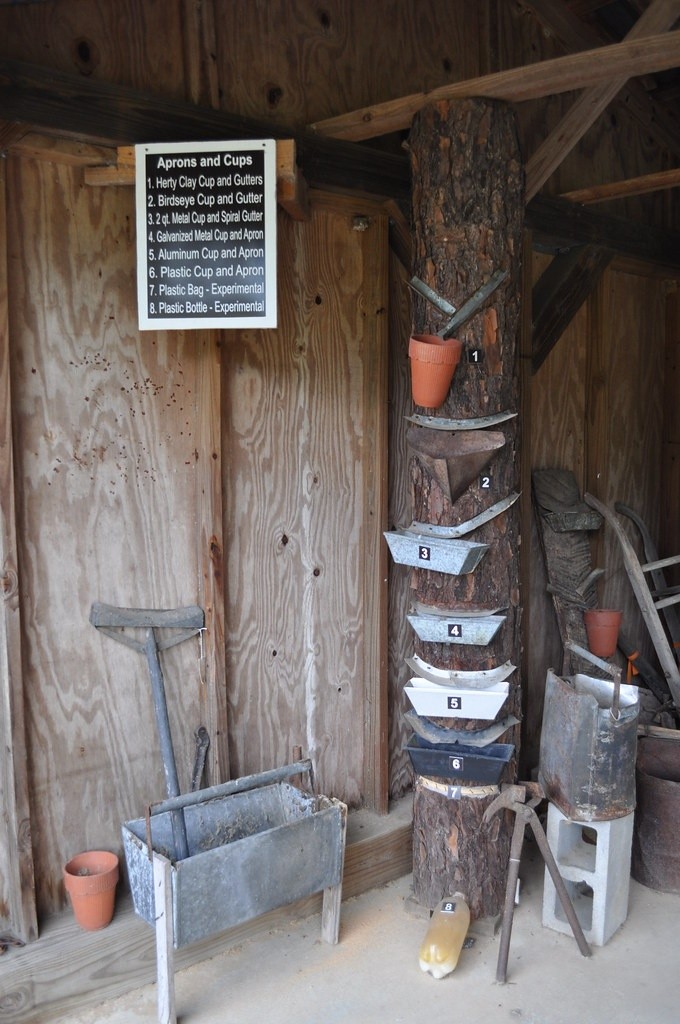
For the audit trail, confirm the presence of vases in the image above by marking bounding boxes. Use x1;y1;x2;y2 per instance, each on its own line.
405;427;505;507
408;335;463;409
403;677;510;720
406;611;508;646
545;511;604;532
401;733;516;785
583;608;624;657
382;530;490;575
62;850;120;931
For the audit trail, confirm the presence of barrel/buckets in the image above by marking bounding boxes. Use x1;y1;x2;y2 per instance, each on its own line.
536;637;643;822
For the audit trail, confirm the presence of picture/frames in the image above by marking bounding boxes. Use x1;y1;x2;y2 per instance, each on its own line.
134;139;281;332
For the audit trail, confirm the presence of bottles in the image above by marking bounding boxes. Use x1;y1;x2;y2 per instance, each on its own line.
418;891;471;979
